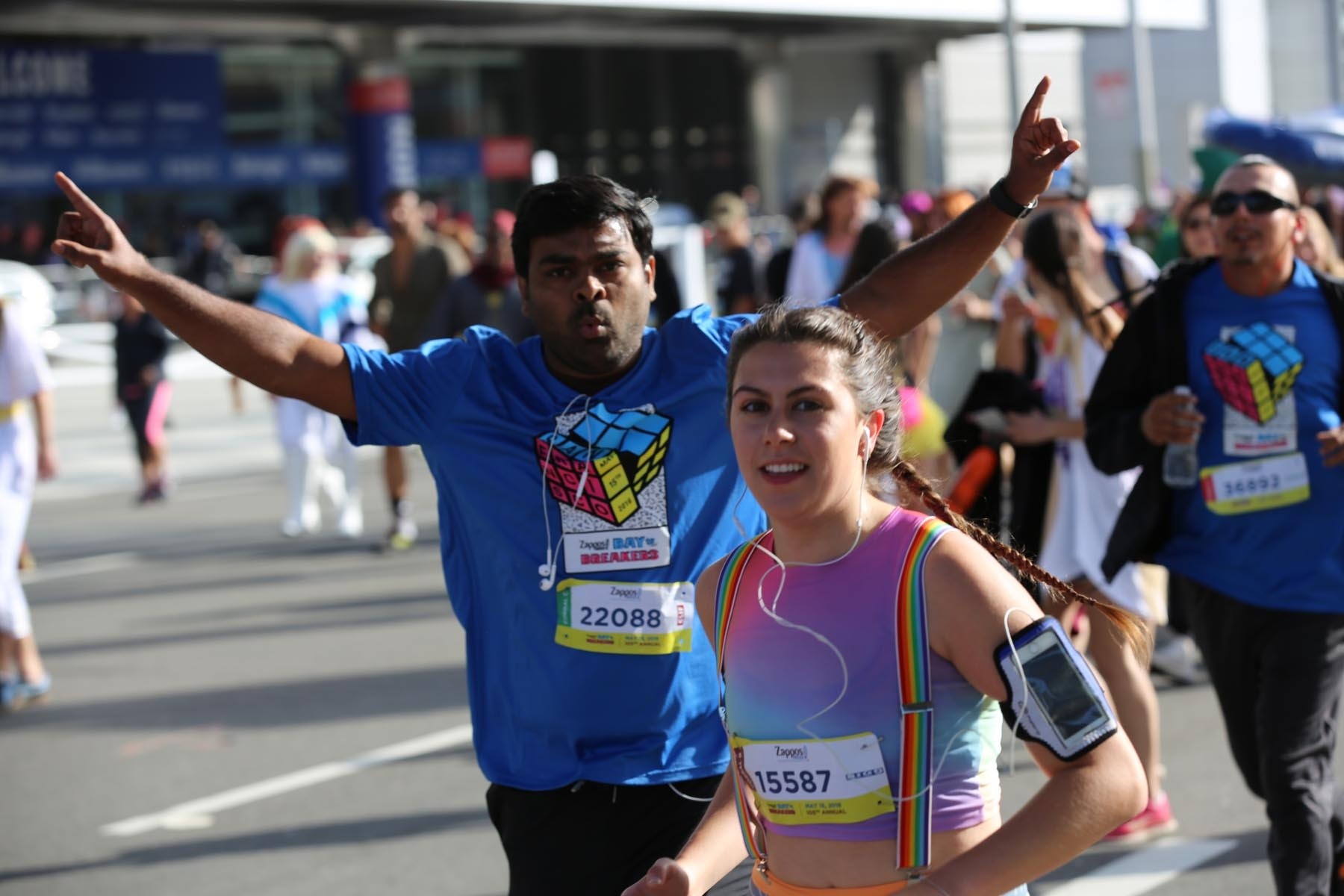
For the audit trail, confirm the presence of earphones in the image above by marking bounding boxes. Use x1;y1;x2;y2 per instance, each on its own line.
859;426;872;461
537;548;558;592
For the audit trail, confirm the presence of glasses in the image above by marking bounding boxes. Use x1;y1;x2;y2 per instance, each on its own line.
1209;189;1299;217
1183;217;1210;232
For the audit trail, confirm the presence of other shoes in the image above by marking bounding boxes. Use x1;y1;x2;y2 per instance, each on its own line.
1151;636;1208;682
1105;791;1178;843
138;482;165;504
339;513;365;537
388;517;419;551
283;510;321;537
1152;624;1182;649
1;674;50;714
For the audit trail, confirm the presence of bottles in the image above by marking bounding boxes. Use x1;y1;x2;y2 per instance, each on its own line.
1163;385;1197;487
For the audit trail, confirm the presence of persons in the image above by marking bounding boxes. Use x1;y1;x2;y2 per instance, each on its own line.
54;75;1079;896
619;307;1151;896
641;176;1344;854
113;292;173;502
188;190;540;548
0;259;57;712
1079;152;1344;896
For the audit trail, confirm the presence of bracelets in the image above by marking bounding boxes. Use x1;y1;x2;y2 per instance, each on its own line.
921;877;949;896
990;178;1038;219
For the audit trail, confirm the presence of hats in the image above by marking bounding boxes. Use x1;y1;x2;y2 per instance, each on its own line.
1038;165;1090;202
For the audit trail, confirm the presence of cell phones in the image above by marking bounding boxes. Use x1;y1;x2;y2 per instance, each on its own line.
1009;627;1112;749
